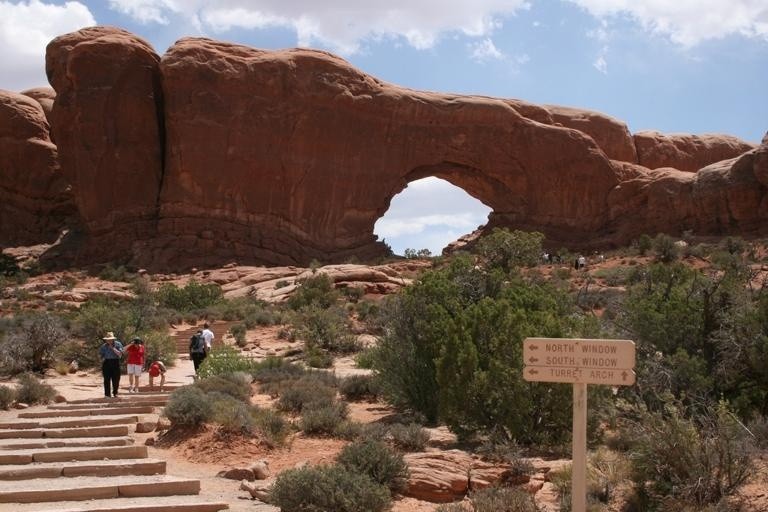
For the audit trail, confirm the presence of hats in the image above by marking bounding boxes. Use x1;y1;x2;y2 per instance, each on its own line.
130;337;143;344
150;365;159;377
102;332;117;341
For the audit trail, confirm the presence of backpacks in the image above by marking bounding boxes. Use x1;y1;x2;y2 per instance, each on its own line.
191;333;203;350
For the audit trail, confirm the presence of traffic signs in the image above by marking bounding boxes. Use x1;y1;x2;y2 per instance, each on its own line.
523;366;635;386
524;336;634;369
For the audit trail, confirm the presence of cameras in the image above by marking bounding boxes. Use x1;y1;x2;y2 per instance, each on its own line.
134;340;142;344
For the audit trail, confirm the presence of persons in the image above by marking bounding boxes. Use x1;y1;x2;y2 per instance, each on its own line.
123;337;146;393
189;330;209;372
202;322;215;358
542;251;601;268
97;331;124;399
148;361;166;392
